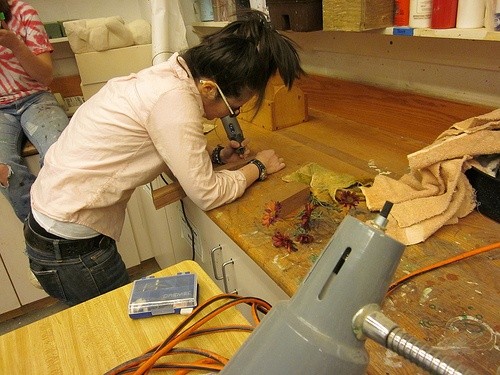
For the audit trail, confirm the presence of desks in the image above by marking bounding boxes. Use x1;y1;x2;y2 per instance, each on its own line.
0;259;255;375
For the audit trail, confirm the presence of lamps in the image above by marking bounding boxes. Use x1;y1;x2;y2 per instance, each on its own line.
218;200;481;375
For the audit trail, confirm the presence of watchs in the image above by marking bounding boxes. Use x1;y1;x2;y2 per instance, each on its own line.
247;159;267;182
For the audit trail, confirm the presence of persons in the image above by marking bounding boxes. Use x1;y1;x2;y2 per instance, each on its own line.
0;0;69;222
23;8;307;307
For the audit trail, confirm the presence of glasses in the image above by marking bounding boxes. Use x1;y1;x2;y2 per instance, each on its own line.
200;80;241;118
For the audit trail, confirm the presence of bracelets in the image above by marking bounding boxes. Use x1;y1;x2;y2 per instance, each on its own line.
211;146;225;165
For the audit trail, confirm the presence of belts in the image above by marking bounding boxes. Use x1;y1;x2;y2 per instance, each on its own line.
23;219;110;254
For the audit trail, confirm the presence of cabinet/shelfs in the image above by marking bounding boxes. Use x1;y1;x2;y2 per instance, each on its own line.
0;45;290;328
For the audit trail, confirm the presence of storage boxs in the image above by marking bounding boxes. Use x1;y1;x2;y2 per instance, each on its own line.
322;0;395;31
128;271;198;318
